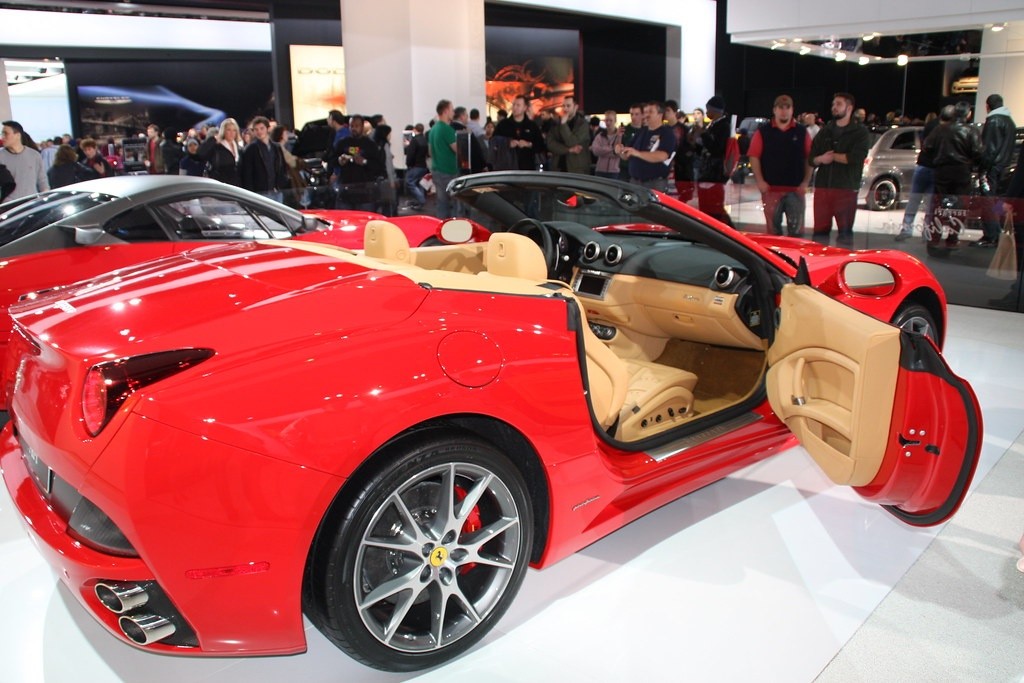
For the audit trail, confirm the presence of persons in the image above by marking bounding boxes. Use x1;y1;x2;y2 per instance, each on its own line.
746;95;814;239
923;101;984;249
809;92;868;250
895;105;954;243
0;94;749;227
969;94;1015;247
794;110;938;134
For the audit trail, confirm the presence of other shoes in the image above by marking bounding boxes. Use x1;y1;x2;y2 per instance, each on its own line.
413;204;425;210
928;239;940;247
895;229;912;241
946;240;961;249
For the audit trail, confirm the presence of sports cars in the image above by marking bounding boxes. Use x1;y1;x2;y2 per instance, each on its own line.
0;174;495;260
0;166;985;673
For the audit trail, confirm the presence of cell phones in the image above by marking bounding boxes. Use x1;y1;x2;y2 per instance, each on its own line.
621;122;624;132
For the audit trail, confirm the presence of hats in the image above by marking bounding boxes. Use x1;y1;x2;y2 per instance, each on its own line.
707;96;726;112
774;96;793;106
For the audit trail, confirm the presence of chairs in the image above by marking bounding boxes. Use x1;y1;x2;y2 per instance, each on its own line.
361;219;697;434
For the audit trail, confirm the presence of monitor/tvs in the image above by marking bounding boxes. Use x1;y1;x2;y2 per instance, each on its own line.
456;132;485;174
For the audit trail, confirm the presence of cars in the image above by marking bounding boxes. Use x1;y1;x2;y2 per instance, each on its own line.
952;75;979;96
740;117;771;137
486;63;574;116
857;126;928;211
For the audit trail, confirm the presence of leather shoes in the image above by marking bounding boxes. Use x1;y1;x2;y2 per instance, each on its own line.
970;238;997;247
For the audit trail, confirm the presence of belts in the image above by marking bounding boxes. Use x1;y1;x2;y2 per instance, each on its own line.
634;177;664;181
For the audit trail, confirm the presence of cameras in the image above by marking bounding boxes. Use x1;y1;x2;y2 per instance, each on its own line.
620;151;628;160
